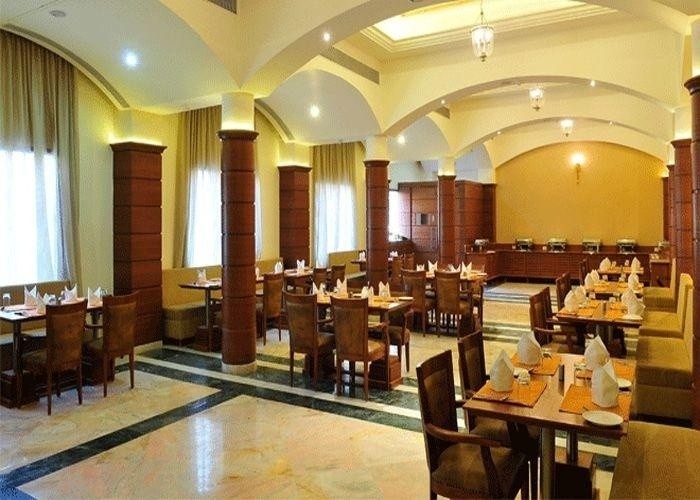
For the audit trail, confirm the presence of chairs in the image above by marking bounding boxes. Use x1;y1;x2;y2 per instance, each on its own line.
386;251;484;339
20;297;90;416
418;349;529;500
457;330;544;500
527;253;694;360
281;249;410;401
255;272;283;346
82;288;143;397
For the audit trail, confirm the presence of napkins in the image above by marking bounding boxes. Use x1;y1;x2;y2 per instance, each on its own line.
197;269;208;285
591;360;620;407
491;351;515;392
517;332;542;364
585;336;610;372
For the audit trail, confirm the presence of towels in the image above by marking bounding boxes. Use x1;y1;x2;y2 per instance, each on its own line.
88;286;101;306
64;284;77;297
24;284;37;303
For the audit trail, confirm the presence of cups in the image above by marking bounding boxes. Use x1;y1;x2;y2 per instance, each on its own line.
574;356;587;367
612;263;615;270
542;344;552;360
519;373;530;386
602;274;608;281
575;369;585;386
381;290;390;305
612;290;620;298
619;277;625;287
2;286;108;308
609;297;616;309
588;291;595;301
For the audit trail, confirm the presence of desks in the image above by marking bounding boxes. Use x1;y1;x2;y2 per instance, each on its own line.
178;276;263;351
463;353;637;500
0;298;103;409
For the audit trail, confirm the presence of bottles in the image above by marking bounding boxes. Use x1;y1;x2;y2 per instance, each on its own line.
602;300;607;310
558;361;565;381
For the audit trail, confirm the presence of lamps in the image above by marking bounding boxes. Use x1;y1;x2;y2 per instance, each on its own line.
528;82;546;111
469;0;497;64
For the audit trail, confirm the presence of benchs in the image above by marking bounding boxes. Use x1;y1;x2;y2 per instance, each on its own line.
0;279;99;398
631;289;695;422
608;424;699;500
161;266;222;345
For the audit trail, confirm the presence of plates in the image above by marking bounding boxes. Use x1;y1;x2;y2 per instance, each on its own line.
477;273;488;276
353;294;361;297
512;366;527;377
615;377;631;390
582;409;624;426
623;316;642;321
398;296;414;301
